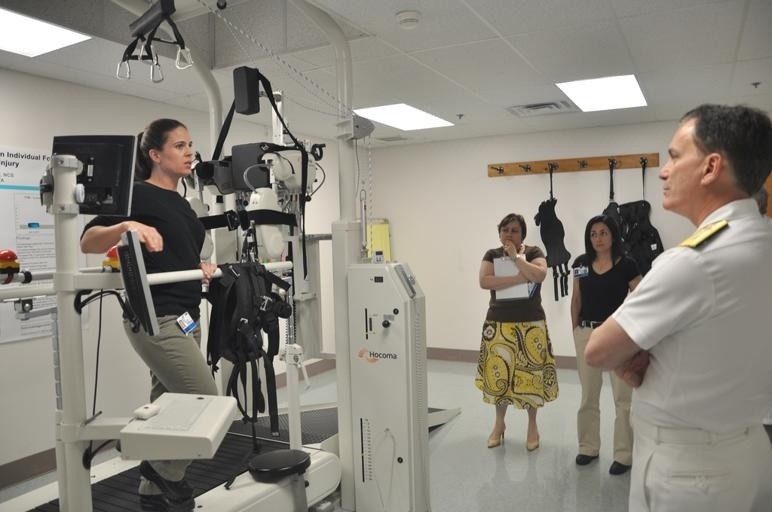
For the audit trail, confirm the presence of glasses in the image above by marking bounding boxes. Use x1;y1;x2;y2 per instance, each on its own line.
590;232;608;238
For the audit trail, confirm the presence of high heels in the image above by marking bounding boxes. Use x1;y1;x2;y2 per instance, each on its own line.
527;430;539;451
487;425;506;448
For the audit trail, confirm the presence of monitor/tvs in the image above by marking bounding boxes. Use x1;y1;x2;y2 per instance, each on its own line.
117;231;160;337
52;136;138;218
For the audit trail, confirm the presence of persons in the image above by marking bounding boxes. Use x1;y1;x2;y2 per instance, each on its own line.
584;104;772;512
80;118;218;512
571;215;643;474
479;213;559;450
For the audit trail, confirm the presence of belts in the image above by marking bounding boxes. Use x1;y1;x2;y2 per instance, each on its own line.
629;418;749;446
577;318;607;330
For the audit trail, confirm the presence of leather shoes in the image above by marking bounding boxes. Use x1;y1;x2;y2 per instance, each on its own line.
576;453;599;465
138;460;195;512
609;461;631;475
